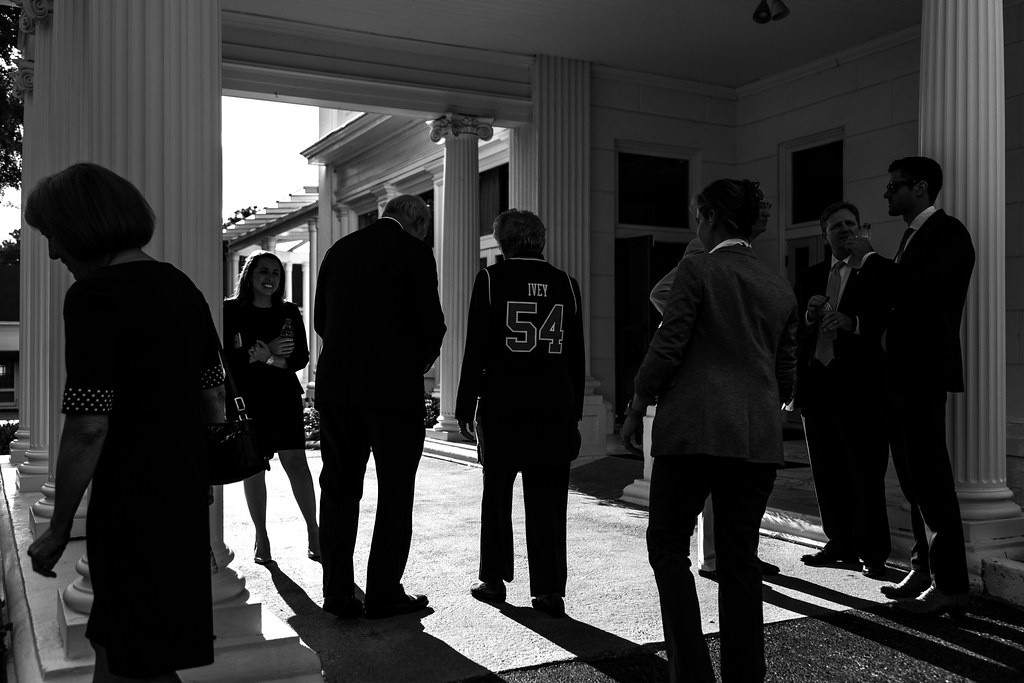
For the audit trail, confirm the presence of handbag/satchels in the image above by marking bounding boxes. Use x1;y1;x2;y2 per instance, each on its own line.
202;418;270;485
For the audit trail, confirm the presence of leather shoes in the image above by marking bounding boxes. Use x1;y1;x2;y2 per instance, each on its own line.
896;588;965;619
882;569;931;599
862;562;885;575
801;548;859;567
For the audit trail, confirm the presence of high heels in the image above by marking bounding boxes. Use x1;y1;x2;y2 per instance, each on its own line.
255;537;271;562
308;538;321;557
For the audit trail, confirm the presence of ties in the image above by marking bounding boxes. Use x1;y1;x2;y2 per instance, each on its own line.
897;228;915;263
814;261;846;366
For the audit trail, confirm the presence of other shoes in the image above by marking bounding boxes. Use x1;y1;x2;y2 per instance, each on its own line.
471;583;507;601
760;563;780;573
322;595;362;611
533;596;565;617
363;594;429;618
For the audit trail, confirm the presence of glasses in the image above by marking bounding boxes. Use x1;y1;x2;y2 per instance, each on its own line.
887;181;920;191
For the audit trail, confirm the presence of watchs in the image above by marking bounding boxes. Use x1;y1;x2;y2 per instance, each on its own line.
624;399;647;417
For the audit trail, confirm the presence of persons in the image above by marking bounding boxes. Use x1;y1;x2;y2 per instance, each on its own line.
223;252;320;563
25;160;226;683
792;156;975;613
314;194;447;620
455;208;587;616
621;181;798;683
